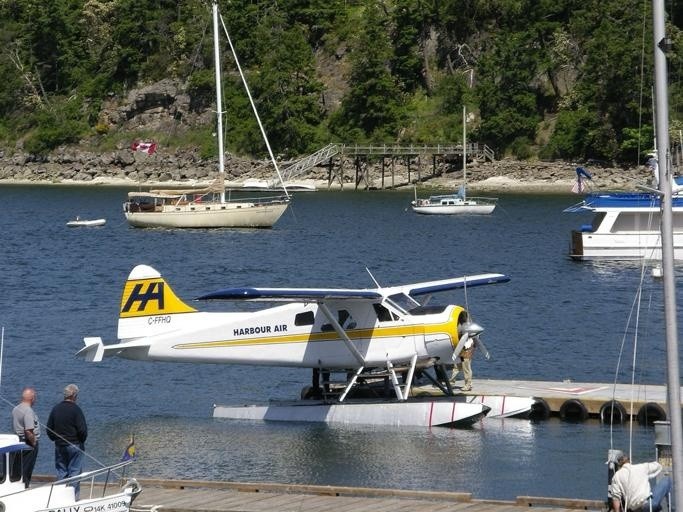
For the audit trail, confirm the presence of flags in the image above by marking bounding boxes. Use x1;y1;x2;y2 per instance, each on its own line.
130;140;156;155
570;175;585;194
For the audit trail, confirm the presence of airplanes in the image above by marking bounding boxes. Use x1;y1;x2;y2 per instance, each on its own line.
75;265;537;428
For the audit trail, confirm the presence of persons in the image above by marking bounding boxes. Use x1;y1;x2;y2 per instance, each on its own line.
606;448;673;512
9;384;91;499
449;333;475;391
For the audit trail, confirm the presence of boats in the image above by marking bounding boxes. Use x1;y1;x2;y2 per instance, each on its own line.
411;105;499;214
66;218;106;228
122;4;291;228
0;425;143;512
563;177;683;262
